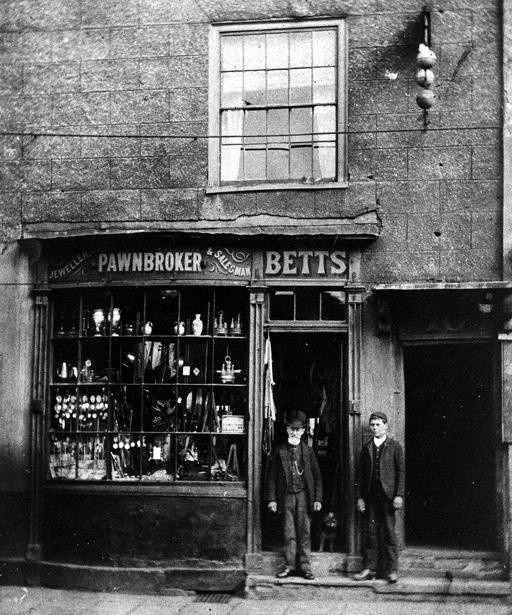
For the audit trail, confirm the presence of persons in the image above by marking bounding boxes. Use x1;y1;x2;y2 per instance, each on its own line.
263;410;324;580
353;410;406;583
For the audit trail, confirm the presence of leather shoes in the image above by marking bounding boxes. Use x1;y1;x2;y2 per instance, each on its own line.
354;569;398;583
277;568;315;579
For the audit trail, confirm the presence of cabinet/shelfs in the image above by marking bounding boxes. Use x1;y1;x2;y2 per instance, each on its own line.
45;286;250;487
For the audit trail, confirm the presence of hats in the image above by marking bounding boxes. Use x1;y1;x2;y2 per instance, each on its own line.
287;410;307;428
370;413;386;419
